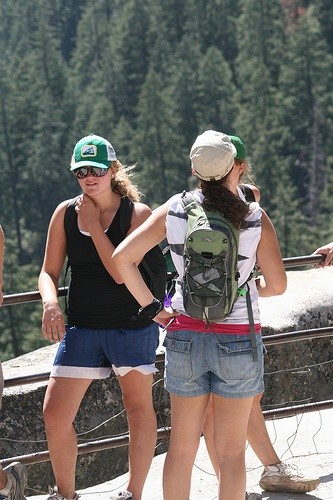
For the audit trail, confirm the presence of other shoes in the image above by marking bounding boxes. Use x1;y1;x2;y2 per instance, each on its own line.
245;492;261;500
0;462;27;500
46;487;80;500
108;490;132;500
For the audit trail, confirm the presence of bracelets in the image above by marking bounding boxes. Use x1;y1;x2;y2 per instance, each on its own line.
138;298;164;322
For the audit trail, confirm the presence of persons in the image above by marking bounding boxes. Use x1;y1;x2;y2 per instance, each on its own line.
112;130;320;500
38;133;166;500
0;226;27;500
311;242;333;268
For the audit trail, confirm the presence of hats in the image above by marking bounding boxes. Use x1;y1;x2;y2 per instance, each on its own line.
68;134;116;169
189;130;244;181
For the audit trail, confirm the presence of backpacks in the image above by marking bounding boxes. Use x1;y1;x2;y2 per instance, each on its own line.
178;191;258;365
64;194;169;309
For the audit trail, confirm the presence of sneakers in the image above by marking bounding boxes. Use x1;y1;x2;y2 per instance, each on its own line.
260;462;320;494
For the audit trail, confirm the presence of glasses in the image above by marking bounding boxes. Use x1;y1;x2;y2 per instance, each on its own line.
73;166;111;179
